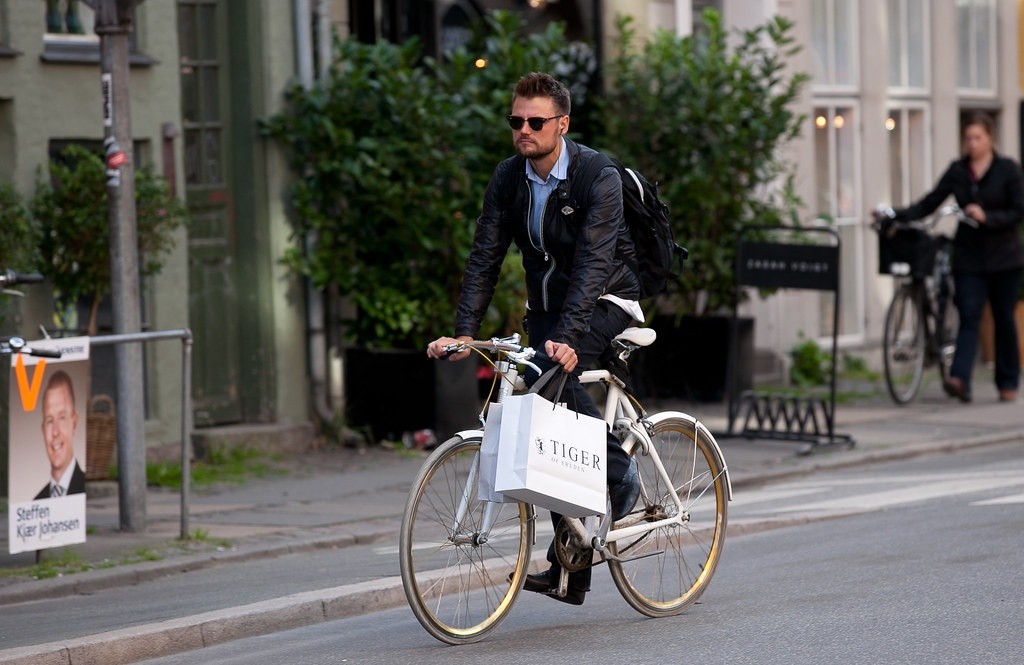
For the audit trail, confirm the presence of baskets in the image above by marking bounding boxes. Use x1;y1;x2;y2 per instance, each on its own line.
879;222;932;276
86;395;118;479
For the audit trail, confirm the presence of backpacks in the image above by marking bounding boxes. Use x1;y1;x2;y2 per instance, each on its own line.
510;154;688;299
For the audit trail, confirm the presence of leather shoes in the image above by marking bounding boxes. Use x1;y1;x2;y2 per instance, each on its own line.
609;459;640;521
510;566;585;606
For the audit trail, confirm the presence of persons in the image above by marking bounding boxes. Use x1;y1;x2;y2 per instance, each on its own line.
428;70;642;606
34;370;84;500
874;113;1024;400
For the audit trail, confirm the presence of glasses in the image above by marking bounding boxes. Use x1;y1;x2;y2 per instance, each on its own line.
505;113;563;131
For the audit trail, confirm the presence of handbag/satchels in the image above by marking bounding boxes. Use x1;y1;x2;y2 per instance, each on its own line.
478;362;610;518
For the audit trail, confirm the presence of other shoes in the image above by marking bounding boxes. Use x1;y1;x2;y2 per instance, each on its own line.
946;378;971;402
1000;389;1016;404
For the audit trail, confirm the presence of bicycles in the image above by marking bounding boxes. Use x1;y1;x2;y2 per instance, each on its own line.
399;327;734;645
870;203;959;407
0;270;62;358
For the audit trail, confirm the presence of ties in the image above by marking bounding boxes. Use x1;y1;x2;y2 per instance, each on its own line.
49;485;65;498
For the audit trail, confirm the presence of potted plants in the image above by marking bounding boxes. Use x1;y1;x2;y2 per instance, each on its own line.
595;4;815;407
257;42;503;442
